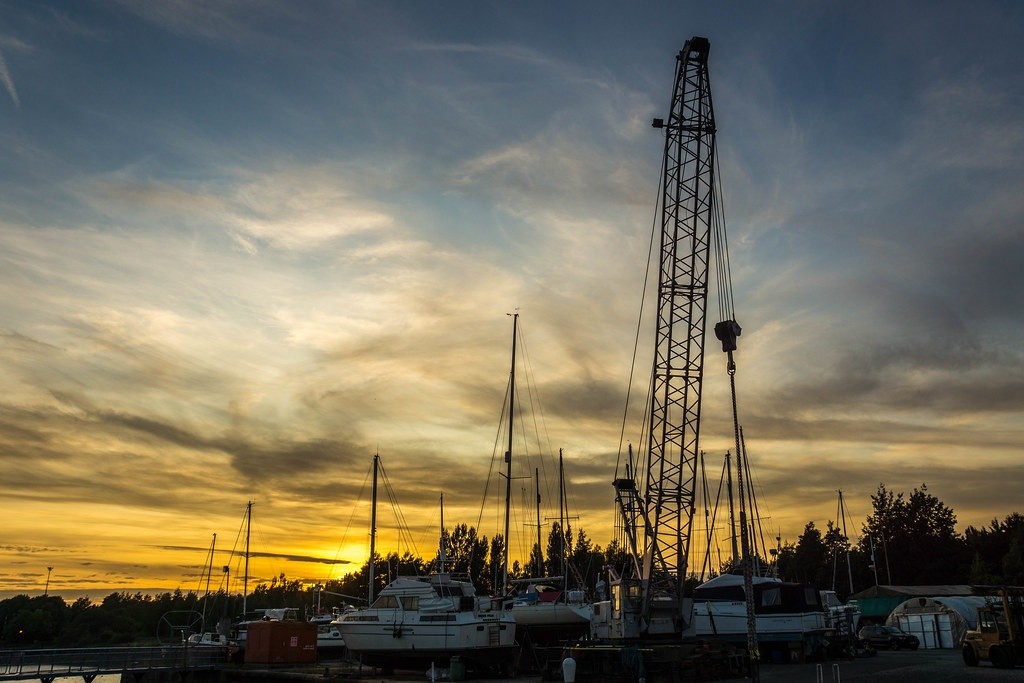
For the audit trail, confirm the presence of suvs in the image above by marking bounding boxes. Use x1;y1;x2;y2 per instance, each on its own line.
858;624;920;652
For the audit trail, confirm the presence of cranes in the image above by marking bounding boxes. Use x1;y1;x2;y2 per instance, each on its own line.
612;34;749;626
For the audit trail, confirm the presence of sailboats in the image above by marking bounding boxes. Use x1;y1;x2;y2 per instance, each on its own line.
182;308;849;661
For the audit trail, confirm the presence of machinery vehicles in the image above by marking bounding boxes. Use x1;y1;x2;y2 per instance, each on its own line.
957;583;1024;670
